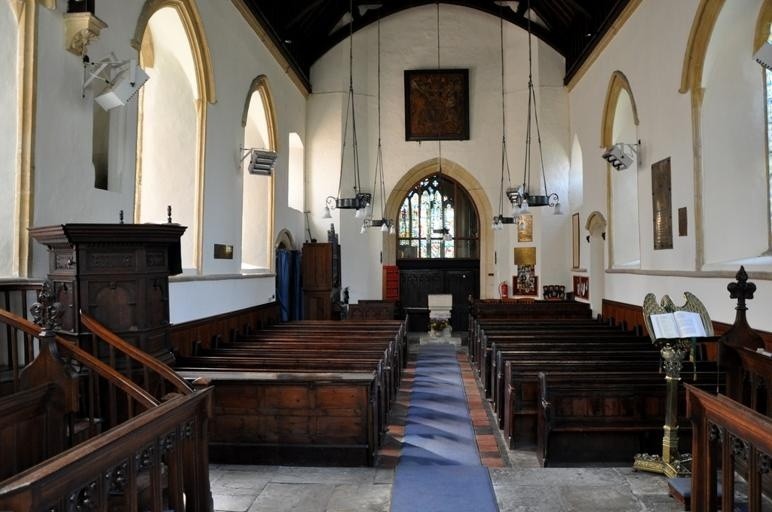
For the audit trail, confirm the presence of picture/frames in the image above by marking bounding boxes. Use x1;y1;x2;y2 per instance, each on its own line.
402;67;469;140
649;156;675;251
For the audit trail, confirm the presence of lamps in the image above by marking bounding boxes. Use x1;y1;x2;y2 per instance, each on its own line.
506;186;524;207
602;142;641;171
511;0;564;216
83;57;151;114
321;1;371;219
239;145;277;177
360;0;397;236
491;0;526;231
426;1;452;241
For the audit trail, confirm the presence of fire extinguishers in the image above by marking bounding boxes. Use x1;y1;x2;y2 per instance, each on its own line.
498;281;509;298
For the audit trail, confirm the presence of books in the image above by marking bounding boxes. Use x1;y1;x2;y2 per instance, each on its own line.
649;310;707;340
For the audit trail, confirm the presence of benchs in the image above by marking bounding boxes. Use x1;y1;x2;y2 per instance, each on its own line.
537;372;717;467
159;368;383;467
504;360;716;450
496;349;697;430
479;323;643;391
490;340;709;414
468;313;603;364
471;315;615;369
274;318;408;352
250;329;404;377
208;346;393;426
260;323;406;370
473;320;627;375
222;341;397;410
187;356;391;449
238;335;401;397
483;334;652;400
